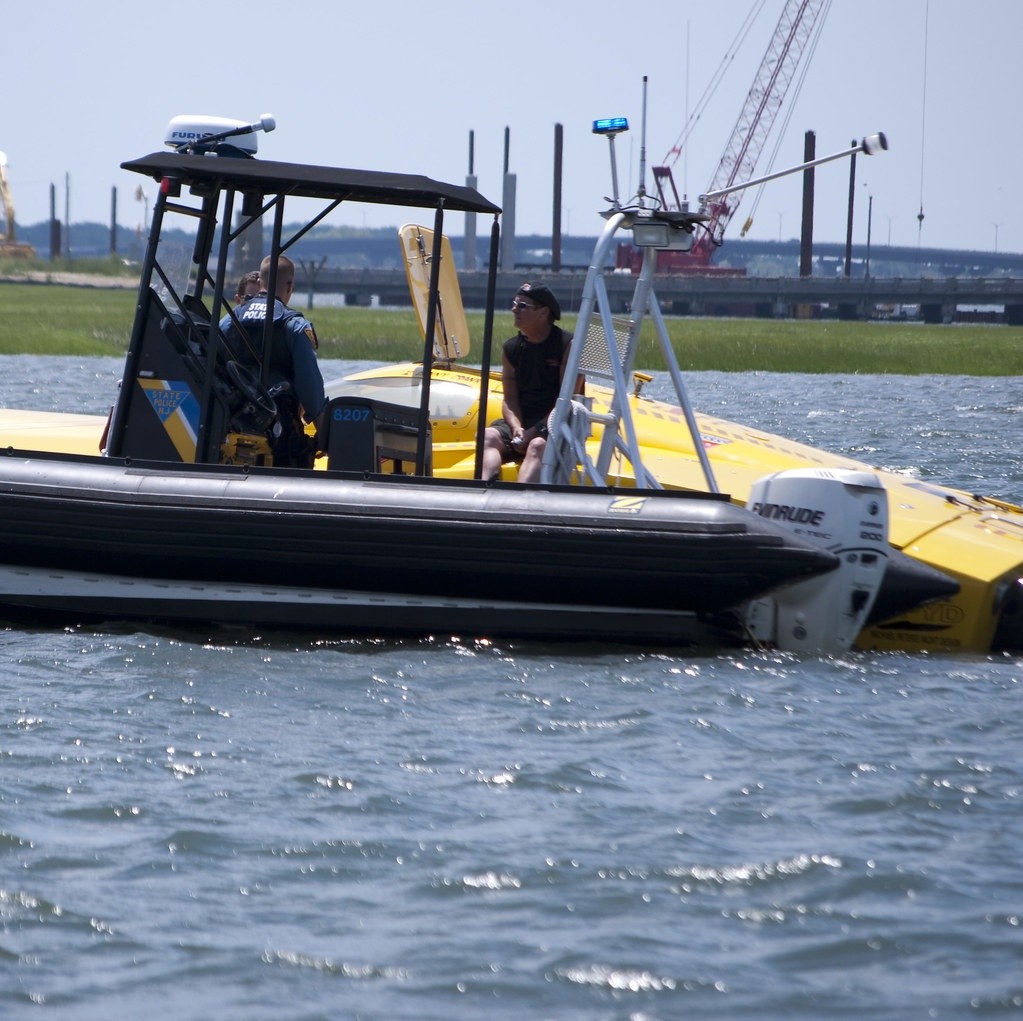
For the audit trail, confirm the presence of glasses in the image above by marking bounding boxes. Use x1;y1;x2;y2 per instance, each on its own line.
240;294;253;301
512;301;540;311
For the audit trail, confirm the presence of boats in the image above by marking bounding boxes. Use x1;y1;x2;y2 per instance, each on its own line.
0;222;1022;654
0;77;962;659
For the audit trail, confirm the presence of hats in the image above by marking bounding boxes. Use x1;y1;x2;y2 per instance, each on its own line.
517;281;561;321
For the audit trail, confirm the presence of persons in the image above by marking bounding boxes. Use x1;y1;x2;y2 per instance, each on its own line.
232;254;327;469
217;271;261;346
475;281;575;482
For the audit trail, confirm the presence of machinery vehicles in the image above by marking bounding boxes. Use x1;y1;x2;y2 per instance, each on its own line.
611;0;835;275
